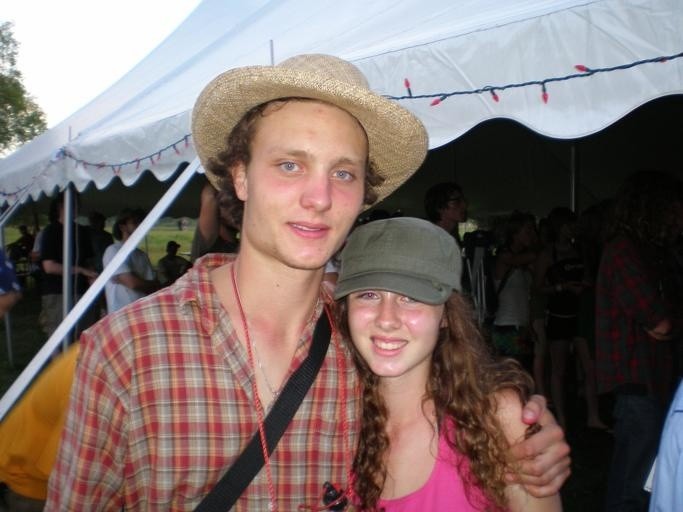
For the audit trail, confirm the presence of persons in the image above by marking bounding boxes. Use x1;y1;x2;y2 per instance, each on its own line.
41;52;575;512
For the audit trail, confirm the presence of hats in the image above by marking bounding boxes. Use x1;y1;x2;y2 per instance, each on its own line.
189;54;429;214
332;217;463;306
